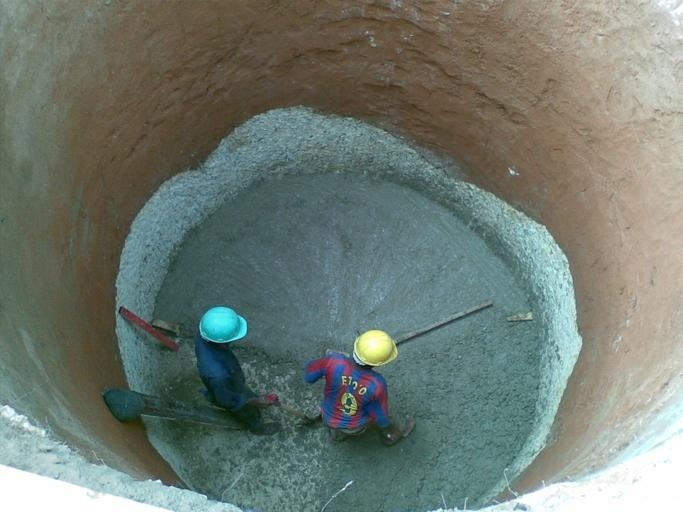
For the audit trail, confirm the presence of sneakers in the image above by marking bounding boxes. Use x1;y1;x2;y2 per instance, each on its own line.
251;423;279;436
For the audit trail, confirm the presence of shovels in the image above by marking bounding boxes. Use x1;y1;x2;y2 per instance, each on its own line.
264;394;323;427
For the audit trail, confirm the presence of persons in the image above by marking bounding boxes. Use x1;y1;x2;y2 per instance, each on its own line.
193;306;279;436
303;329;415;445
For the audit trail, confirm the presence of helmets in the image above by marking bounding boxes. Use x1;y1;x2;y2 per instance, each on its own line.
199;307;247;344
354;330;398;366
